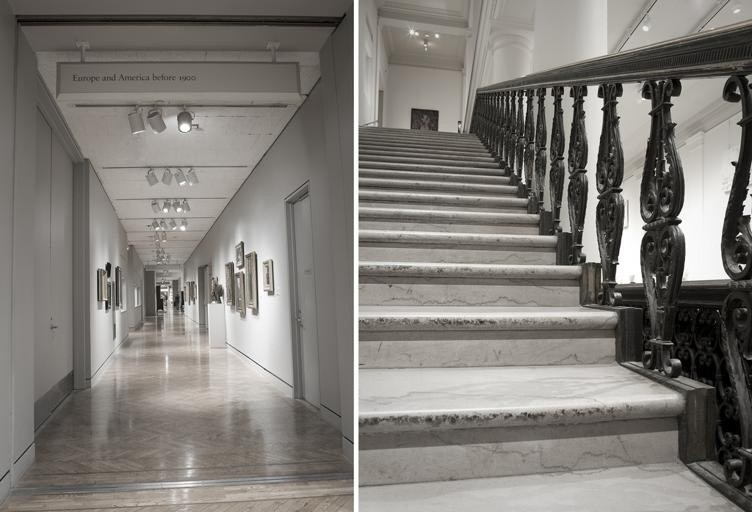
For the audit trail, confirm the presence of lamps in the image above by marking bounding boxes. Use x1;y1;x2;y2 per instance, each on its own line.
151;199;191;215
126;103;200;137
145;168;199;187
148;218;188;244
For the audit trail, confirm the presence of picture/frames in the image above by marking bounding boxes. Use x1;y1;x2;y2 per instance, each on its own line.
224;241;258;319
97;262;123;311
261;258;274;292
410;107;440;131
